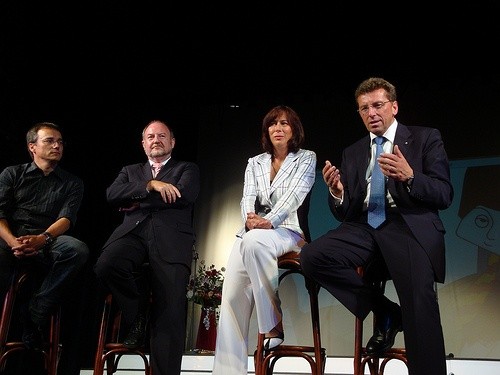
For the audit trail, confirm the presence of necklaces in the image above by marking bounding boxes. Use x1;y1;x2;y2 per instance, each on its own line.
271;162;276;174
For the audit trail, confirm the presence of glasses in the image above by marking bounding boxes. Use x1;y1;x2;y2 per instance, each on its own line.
357;101;392;111
42;139;62;146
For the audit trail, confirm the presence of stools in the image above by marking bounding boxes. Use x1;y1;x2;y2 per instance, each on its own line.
0;259;62;375
254;251;328;375
351;257;409;375
93;262;159;375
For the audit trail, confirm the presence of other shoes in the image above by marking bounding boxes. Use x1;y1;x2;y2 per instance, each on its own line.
261;321;283;350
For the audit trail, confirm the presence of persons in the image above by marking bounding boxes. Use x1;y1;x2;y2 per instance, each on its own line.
300;77;455;375
96;119;197;375
212;106;316;375
0;122;88;352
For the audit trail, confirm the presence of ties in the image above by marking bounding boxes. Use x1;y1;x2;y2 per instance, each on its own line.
368;137;388;229
153;163;163;178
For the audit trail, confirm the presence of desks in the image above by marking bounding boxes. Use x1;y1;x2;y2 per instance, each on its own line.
195;303;217;353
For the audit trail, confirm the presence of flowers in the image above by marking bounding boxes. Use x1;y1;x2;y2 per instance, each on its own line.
186;260;224;331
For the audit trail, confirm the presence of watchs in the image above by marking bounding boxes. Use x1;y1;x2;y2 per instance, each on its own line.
405;177;414;188
42;231;54;244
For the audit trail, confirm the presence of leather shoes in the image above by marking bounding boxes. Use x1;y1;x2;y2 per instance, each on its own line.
123;321;149;346
366;303;402;355
23;319;45;350
